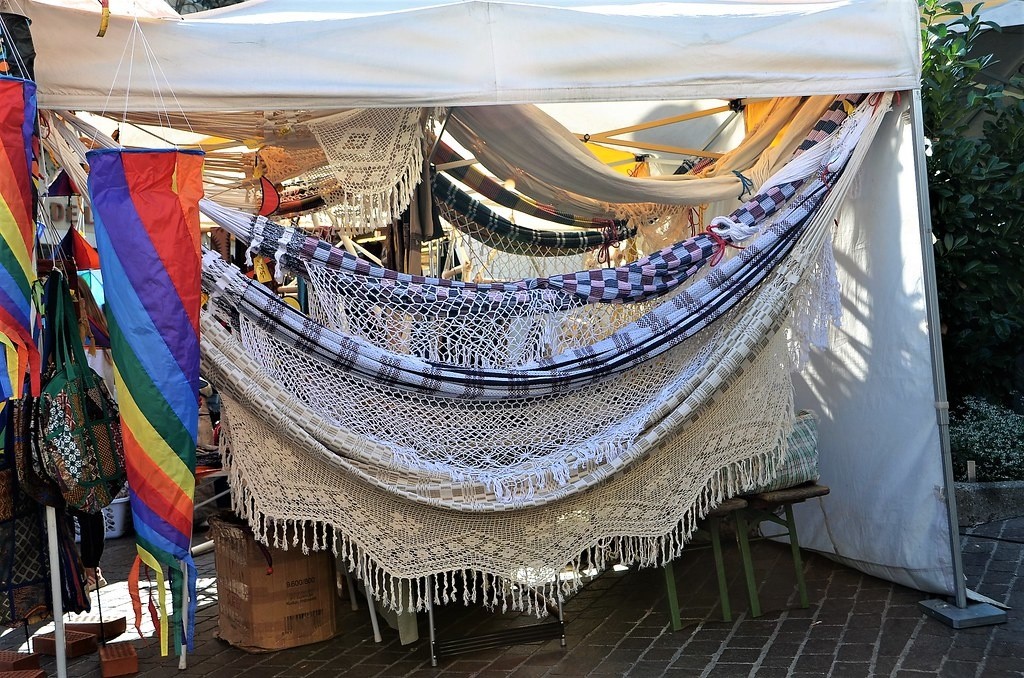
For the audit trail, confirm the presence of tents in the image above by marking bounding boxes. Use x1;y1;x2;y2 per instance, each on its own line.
0;0;973;607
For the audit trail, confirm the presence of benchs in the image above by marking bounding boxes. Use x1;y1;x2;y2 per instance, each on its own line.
645;485;830;633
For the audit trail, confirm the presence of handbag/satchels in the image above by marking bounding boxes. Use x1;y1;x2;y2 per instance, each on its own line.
707;409;821;495
11;269;126;620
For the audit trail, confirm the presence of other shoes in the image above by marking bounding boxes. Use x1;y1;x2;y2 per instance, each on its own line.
86;575;107;590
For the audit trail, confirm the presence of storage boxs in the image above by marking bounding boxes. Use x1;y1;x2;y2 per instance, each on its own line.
72;496;131;543
206;518;339;654
